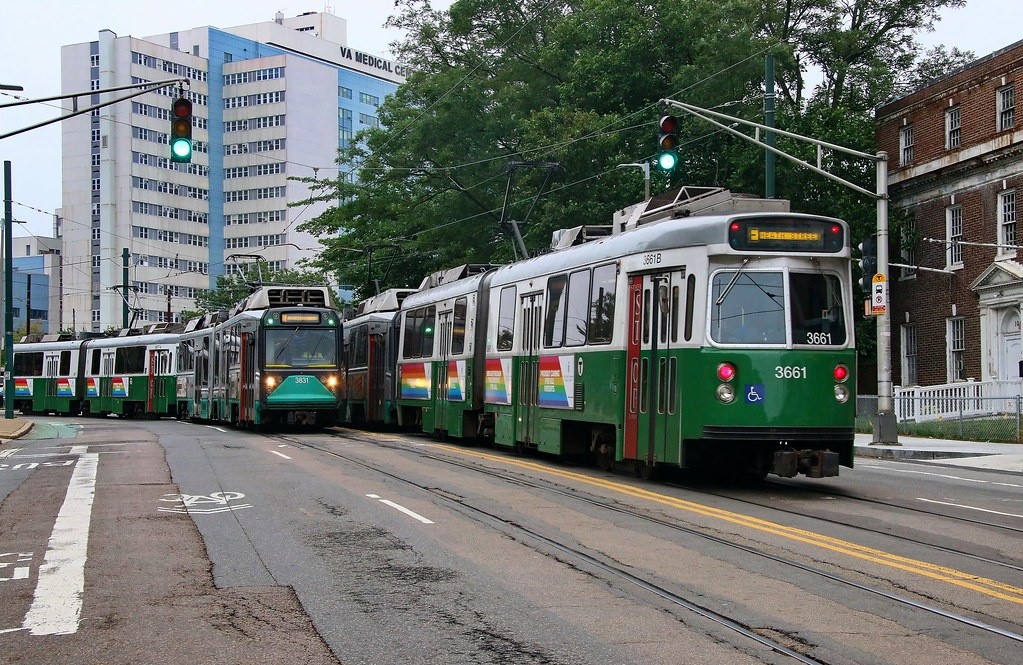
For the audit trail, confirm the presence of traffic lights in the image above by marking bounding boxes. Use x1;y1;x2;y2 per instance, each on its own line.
656;114;681;172
858;237;877;292
169;97;194;164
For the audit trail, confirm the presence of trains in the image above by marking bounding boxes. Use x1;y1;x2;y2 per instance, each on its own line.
0;282;348;433
341;183;856;488
